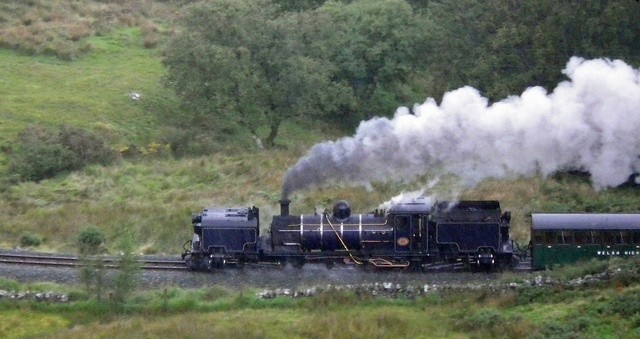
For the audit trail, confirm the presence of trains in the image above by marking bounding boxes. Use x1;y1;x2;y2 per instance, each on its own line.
181;195;639;274
